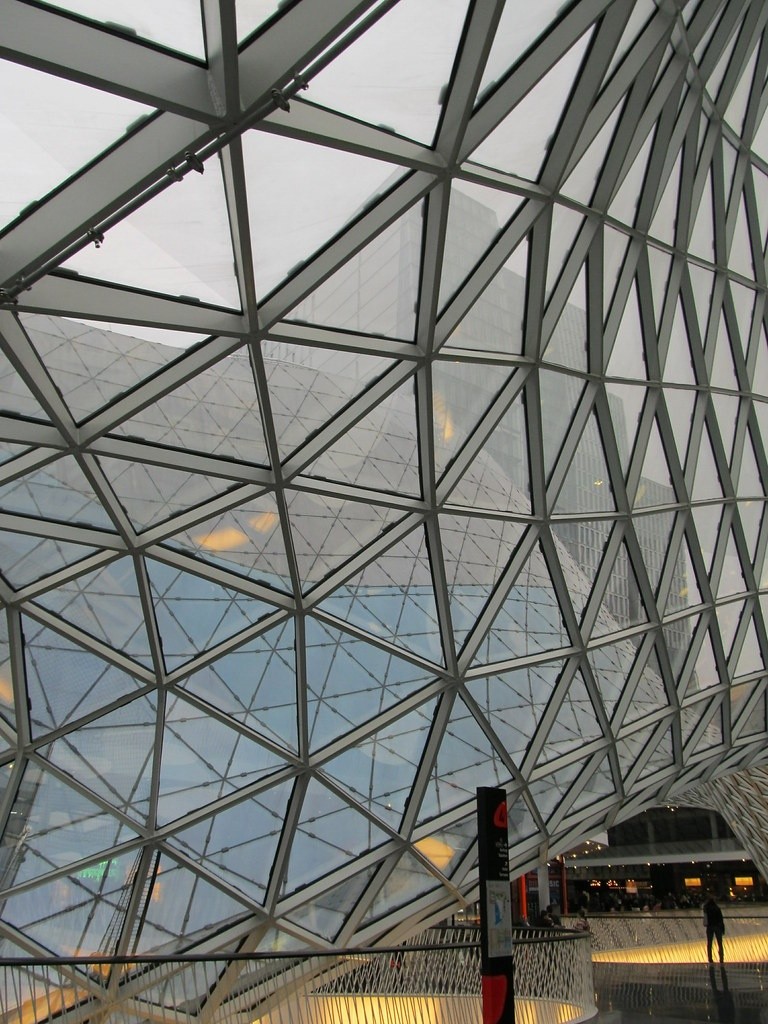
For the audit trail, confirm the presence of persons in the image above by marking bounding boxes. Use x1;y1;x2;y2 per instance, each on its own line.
532;905;563;928
577;889;705;911
703;896;725;963
574;910;590;931
